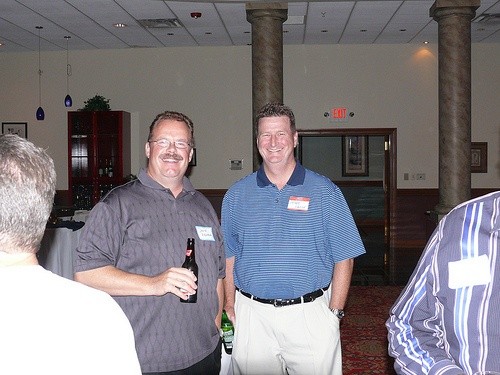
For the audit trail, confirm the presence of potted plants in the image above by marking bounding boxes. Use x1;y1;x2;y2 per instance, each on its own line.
78;95;110;112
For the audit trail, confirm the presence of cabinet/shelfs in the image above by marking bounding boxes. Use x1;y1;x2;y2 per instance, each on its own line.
67;110;132;209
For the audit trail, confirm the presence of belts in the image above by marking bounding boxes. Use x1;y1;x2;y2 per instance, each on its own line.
235;283;330;307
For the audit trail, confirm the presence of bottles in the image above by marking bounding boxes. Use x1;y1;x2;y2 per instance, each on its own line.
98;159;113;178
221;309;236;356
180;238;199;303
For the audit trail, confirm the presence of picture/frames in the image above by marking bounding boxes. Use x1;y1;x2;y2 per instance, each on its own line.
341;136;370;177
1;122;28;139
471;141;488;173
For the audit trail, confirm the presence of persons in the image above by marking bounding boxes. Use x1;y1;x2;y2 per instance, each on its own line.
384;191;500;375
220;101;366;375
73;111;226;375
0;134;142;375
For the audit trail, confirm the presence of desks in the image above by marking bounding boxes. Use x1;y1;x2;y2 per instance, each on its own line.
37;216;84;280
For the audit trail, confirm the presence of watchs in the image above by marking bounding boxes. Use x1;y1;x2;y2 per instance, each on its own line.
329;307;345;319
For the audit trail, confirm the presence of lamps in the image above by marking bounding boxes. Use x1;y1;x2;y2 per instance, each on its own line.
64;36;73;107
324;107;355;123
35;26;45;121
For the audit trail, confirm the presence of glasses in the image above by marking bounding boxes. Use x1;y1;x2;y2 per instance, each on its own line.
149;139;190;149
